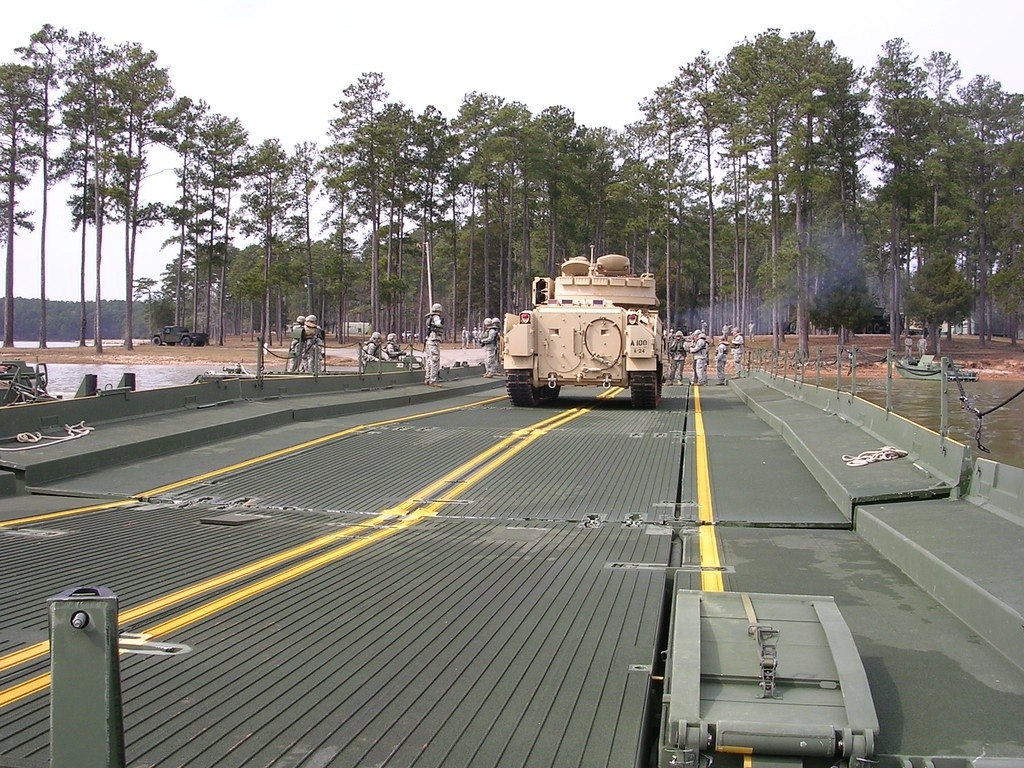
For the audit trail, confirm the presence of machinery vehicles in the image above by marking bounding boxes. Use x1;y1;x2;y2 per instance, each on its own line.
909;319;943;335
857;306;904;333
502;243;668;408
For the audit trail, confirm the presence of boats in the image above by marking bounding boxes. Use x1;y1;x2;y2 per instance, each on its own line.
895;356;979;382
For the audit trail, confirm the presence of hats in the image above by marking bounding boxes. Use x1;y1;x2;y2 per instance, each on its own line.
669;329;674;332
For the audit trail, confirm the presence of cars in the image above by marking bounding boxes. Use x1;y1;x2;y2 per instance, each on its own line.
401;331;418;339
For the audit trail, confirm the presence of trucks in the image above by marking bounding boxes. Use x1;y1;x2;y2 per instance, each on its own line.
341;322;373;335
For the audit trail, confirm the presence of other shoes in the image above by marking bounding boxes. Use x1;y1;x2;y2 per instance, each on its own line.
715;382;724;386
693;382;706;386
430;382;442;387
664;380;673;386
677;379;683;386
479;372;493;378
730;374;741;379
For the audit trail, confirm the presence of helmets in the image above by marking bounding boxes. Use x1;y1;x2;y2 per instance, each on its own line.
483;318;492;325
492;318;500;323
306;315;317;322
733;327;740;332
693;330;702;335
432;303;442;311
697;334;707;339
387;333;397;340
372;332;381;338
296;316;306;323
675;331;683;337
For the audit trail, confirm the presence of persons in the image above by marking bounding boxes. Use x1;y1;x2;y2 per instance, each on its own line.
904;334;913;359
747;320;756;342
461;318;503;378
662;320;744;387
385;333;406;361
917;335;927;358
361;332;386;370
286;314;323;373
424;303;444;387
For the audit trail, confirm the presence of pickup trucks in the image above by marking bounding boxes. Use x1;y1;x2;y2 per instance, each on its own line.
151;325;207;347
